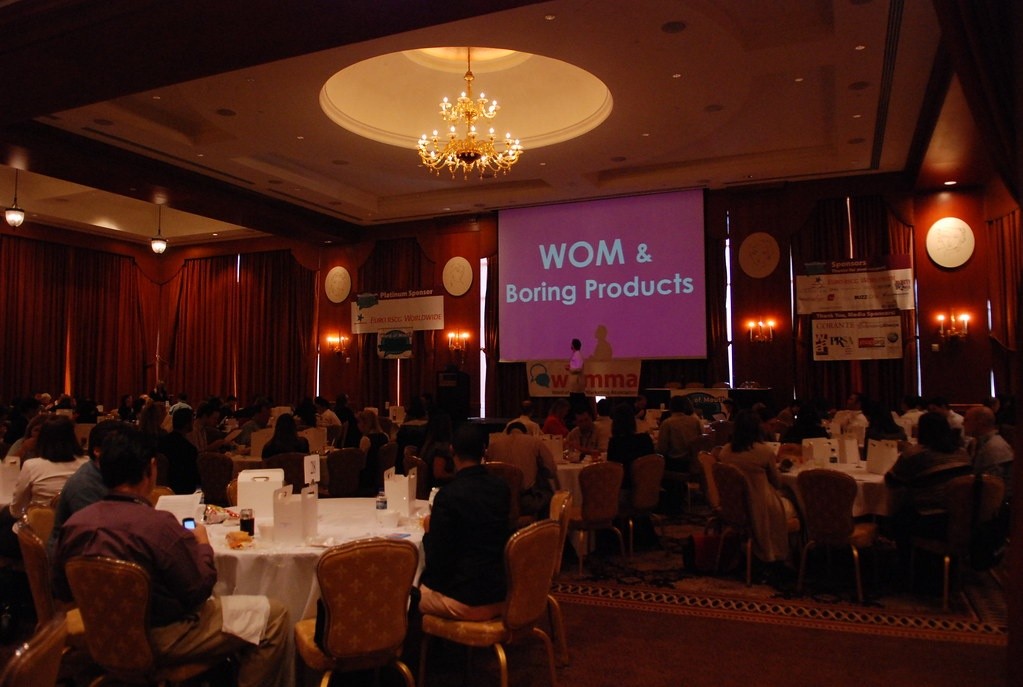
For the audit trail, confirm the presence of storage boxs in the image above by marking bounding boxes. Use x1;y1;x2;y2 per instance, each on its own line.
0;455;21;483
831;433;861;464
272;485;319;544
866;439;900;474
237;469;285;518
801;437;827;467
539;434;563;465
383;465;417;517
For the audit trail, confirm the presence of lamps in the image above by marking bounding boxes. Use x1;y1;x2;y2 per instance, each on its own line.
939;313;968;354
448;332;470;368
326;335;347;361
151;206;169;255
416;47;524;181
5;169;25;232
748;319;774;355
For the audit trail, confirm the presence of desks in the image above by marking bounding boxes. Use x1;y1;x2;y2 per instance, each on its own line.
201;497;430;687
557;453;602;558
223;447;340;488
779;461;892;519
0;470;16;554
644;387;777;419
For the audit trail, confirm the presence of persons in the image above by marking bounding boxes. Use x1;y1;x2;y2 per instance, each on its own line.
564;339;596;422
45;428;241;664
54;420;137;605
410;418;511;623
0;380;1013;646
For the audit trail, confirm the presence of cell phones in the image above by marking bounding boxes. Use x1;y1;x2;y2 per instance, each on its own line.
182;517;196;529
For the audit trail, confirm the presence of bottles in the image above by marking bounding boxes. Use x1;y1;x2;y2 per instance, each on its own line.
375;489;388;526
828;447;839;469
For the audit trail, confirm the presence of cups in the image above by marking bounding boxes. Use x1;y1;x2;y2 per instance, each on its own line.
239;508;255;538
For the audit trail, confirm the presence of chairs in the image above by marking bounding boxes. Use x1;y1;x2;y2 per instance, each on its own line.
0;405;1013;687
685;382;704;388
664;382;682;389
712;382;730;388
739;381;762;388
